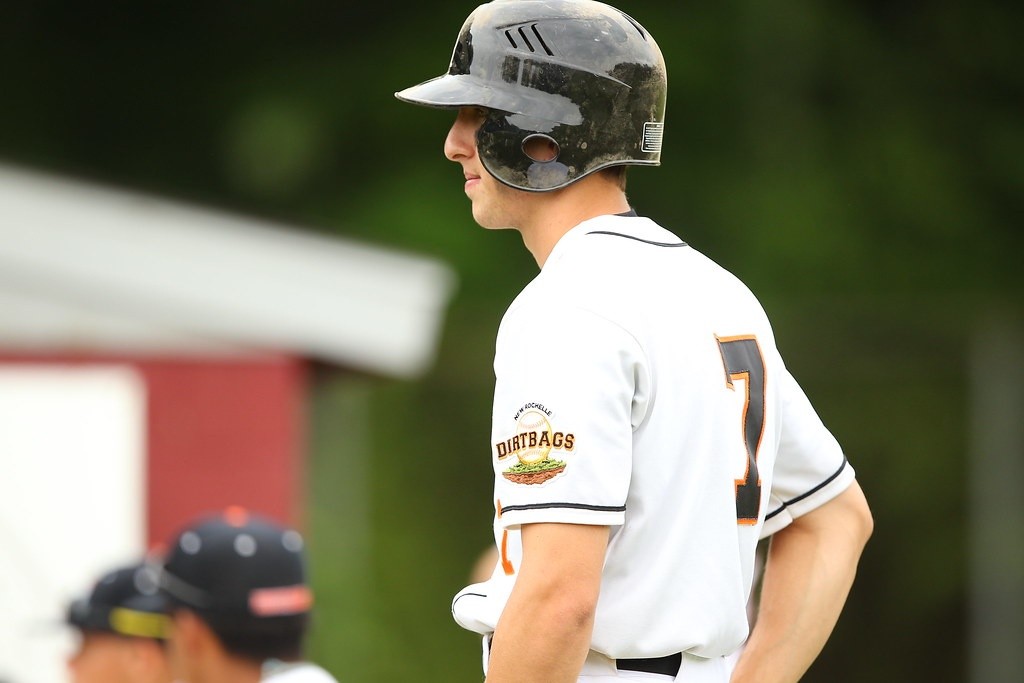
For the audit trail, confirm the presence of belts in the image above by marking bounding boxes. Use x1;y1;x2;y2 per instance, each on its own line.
488;631;682;678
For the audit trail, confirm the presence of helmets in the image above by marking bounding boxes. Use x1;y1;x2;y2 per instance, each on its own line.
394;0;668;193
156;506;316;631
63;563;175;641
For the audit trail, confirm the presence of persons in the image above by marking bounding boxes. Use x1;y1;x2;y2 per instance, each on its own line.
395;0;873;683
66;512;341;683
